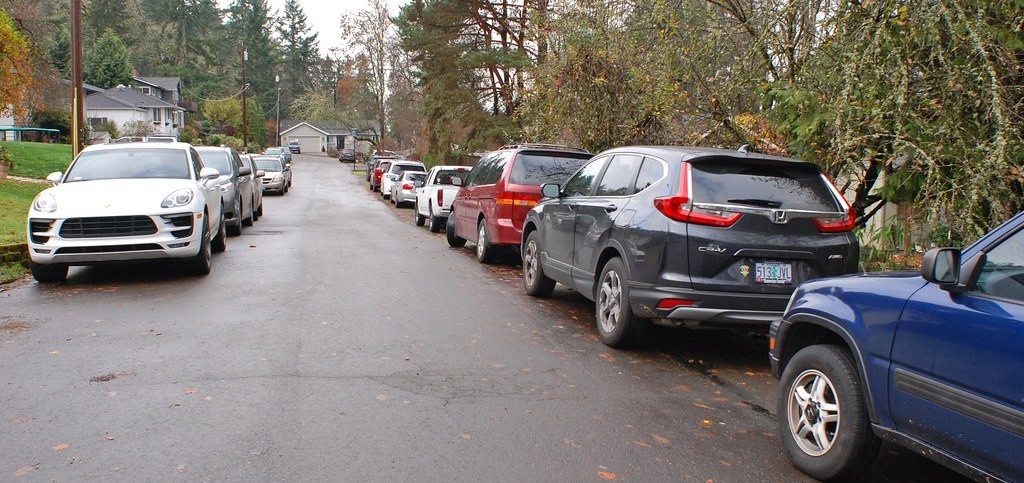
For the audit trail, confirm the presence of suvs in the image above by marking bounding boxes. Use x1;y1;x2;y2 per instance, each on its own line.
444;143;600;264
288;141;301;154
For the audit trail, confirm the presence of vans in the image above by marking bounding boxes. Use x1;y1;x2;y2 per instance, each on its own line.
113;135;179;143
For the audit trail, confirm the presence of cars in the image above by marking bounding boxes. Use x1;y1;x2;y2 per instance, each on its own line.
340;149;356;163
26;142;228;284
191;144;255;237
379;160;427;200
764;206;1024;483
362;149;400;193
239;154;266;220
252;146;292;196
520;144;860;350
389;170;430;208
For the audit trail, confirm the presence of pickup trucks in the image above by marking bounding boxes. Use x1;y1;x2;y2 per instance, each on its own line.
411;166;474;232
